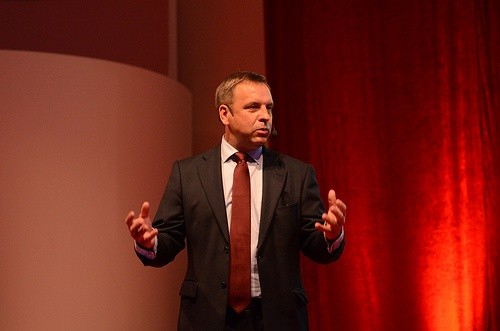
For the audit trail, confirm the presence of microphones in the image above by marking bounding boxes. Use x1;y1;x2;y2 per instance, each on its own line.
272;125;278;136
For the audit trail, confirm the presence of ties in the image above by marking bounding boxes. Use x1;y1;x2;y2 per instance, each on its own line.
227;152;255;313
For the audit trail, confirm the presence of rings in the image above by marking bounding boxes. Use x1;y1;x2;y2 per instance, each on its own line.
141;237;147;243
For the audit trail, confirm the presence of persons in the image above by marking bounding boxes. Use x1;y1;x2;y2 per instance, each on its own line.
125;72;348;331
